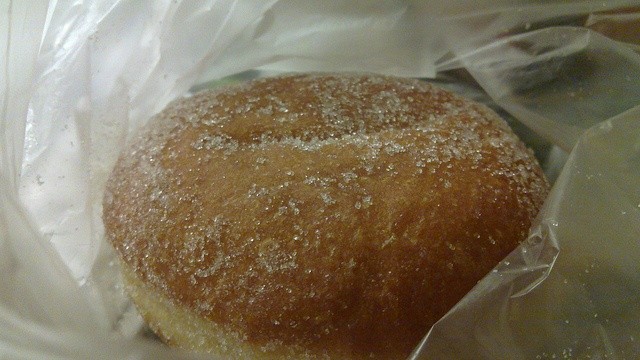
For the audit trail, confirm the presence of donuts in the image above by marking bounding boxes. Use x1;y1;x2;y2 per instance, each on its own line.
101;74;553;360
